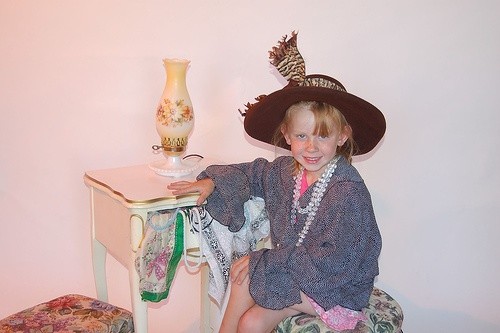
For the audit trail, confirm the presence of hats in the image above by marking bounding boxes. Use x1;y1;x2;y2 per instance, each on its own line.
238;31;386;156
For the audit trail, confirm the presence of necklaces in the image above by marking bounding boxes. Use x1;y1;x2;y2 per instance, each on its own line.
289;160;337;246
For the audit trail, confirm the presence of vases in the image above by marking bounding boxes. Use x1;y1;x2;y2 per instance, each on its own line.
149;58;198;178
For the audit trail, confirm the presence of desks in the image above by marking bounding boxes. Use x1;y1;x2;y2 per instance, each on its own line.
84;161;213;333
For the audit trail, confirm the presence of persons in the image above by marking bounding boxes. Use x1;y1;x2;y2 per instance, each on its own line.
168;73;381;333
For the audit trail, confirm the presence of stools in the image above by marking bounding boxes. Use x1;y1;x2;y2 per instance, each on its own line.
271;287;405;333
0;293;134;333
